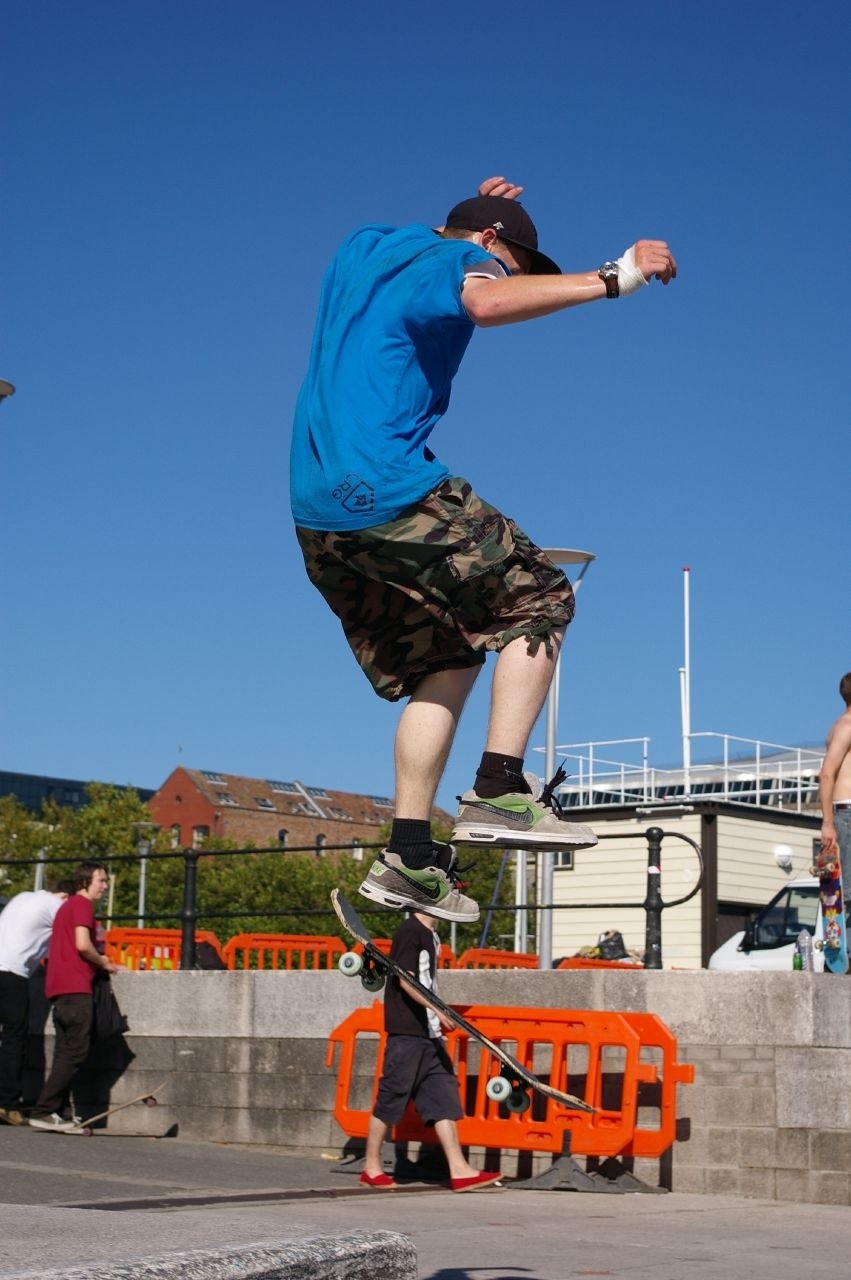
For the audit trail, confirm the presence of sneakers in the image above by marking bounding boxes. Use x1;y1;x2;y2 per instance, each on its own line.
451;757;598;851
28;1113;77;1132
358;838;481;923
61;1117;93;1136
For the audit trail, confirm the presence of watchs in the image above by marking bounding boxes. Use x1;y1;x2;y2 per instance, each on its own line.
598;260;620;299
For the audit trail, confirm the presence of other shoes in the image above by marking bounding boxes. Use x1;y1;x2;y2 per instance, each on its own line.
360;1170;396;1189
452;1171;505;1192
0;1105;30;1126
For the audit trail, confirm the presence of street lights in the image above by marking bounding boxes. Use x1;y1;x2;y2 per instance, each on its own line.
127;822;162;930
537;544;594;969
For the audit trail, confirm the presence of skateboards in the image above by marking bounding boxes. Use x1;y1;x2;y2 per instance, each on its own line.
53;1081;169;1138
326;886;596;1114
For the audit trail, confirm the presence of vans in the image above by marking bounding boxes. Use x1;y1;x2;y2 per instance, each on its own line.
707;876;842;974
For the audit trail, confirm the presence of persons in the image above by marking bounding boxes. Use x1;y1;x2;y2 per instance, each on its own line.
818;672;851;972
0;860;122;1135
358;911;507;1194
289;175;678;924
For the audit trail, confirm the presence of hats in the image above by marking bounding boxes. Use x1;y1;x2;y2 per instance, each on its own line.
446;195;563;274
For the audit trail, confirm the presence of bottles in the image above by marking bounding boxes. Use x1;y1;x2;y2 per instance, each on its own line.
793;952;801;971
797;927;814;970
